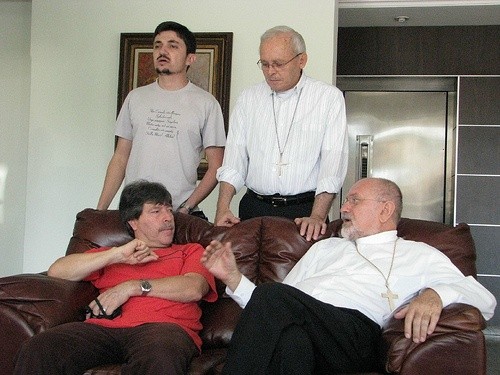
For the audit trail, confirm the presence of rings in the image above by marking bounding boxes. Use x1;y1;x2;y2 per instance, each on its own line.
103;309;107;312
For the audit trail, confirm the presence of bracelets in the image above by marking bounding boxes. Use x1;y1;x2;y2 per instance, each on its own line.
179;203;193;214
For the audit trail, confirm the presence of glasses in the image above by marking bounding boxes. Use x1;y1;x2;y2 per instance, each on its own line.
256;52;304;71
343;197;386;208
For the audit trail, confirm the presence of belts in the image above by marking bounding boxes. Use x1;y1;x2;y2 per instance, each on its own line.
246;187;315;208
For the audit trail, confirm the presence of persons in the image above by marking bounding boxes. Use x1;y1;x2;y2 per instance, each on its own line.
10;178;218;375
96;21;227;214
200;177;497;375
214;25;348;241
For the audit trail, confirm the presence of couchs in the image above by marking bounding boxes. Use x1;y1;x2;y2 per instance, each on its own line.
0;207;487;375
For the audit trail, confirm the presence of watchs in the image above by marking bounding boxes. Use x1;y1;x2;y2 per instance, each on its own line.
140;279;152;296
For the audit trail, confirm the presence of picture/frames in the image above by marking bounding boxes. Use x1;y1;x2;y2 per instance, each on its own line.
114;32;233;180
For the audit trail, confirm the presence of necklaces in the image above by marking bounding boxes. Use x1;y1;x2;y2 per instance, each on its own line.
272;88;303;175
354;237;401;312
